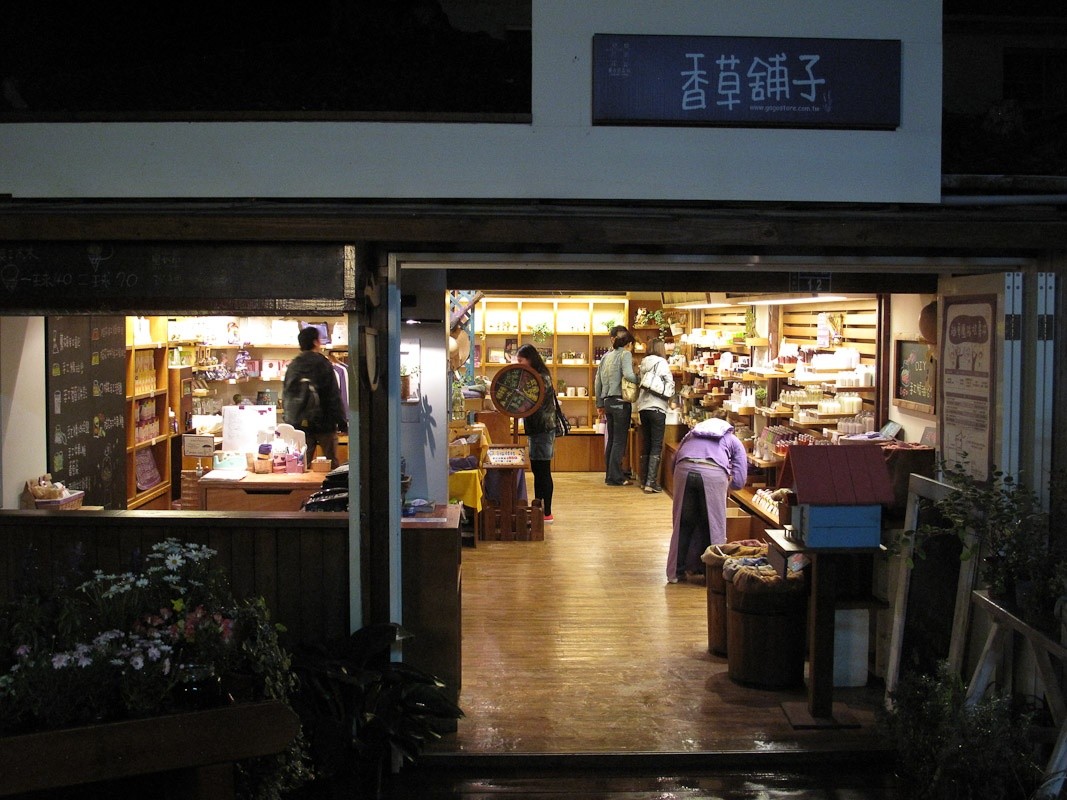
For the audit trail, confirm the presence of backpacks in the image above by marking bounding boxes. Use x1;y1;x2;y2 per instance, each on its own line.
282;358;327;434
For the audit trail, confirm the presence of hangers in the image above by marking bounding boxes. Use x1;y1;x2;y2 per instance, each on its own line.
324;350;342;365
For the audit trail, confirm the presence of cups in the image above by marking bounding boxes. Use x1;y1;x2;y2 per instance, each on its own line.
578;387;587;396
567;387;575;396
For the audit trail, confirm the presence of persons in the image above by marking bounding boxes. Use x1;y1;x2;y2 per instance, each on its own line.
515;343;560;523
282;326;348;470
666;418;747;582
637;337;676;494
594;325;639;486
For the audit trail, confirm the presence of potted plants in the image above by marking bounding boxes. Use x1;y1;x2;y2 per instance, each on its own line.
557;379;566;396
399;364;422;399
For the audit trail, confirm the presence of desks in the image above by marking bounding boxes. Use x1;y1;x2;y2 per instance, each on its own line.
468;421;494;463
198;467;333;512
449;465;486;549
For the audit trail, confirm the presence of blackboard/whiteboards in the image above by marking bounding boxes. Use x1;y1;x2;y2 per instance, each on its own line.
892;333;937;414
44;316;128;509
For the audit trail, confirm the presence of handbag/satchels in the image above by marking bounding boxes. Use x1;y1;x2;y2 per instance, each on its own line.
621;351;640;403
638;359;672;400
555;410;571;437
595;414;606;434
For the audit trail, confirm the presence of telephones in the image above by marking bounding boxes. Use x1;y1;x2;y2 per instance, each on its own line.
304;487;348;511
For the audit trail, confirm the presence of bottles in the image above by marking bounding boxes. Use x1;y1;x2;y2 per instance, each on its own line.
595;347;607;361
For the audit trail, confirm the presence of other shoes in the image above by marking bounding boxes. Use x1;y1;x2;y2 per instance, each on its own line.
668;571;687;584
687;571;706;583
605;474;633;486
623;471;632;479
544;515;554;523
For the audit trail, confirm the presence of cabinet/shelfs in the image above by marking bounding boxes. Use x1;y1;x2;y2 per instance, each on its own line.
125;296;880;542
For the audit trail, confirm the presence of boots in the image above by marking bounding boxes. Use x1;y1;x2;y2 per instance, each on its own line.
645;454;662;493
639;454;649;489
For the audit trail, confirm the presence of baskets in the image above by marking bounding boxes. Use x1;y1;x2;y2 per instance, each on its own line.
34;489;85;510
